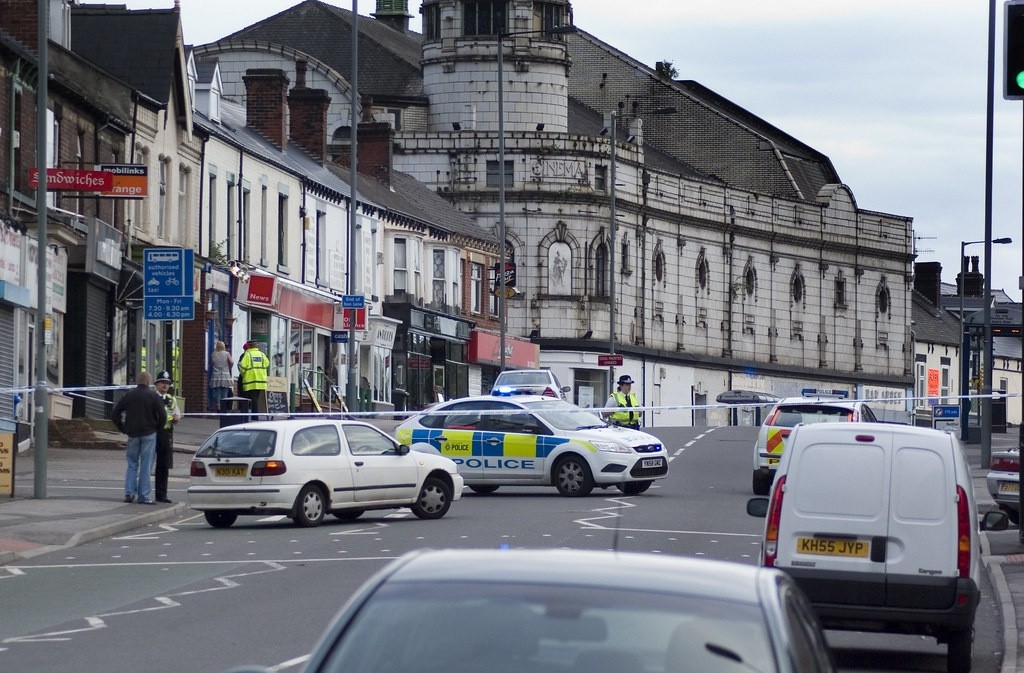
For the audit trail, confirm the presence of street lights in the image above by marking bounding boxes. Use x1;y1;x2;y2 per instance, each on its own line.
609;106;674;401
492;25;579;392
960;237;1012;434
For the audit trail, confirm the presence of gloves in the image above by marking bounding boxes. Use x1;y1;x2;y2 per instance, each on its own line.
160;395;172;407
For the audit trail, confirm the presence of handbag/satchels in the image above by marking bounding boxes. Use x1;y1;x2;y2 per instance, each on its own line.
227;389;234;411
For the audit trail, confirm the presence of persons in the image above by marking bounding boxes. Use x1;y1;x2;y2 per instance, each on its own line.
601;374;644;432
111;372;167;505
236;340;270;423
209;341;234;414
151;370;180;504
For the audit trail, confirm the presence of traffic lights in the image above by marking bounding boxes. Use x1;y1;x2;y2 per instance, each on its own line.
1001;0;1024;101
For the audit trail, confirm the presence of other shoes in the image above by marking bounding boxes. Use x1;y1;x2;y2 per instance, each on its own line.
124;494;135;503
138;497;156;505
156;498;172;503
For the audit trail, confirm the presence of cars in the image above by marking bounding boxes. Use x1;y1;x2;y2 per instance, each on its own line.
184;420;463;527
986;449;1021;526
389;396;669;497
493;369;573;405
298;549;835;673
752;387;880;495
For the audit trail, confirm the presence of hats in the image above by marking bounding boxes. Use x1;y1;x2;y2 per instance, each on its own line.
617;375;634;384
247;340;258;344
243;343;250;351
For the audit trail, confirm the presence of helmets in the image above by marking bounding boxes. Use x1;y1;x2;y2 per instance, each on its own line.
154;370;172;385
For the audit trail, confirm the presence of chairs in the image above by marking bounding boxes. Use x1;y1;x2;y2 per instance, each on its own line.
661;621;774;673
458;600;543;673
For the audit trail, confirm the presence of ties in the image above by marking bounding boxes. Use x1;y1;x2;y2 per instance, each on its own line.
625;395;634;422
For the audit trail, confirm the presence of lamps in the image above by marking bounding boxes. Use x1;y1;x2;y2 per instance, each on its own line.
536;123;544;131
599;128;608;135
204;260;250;284
452;122;461;131
627;135;635;143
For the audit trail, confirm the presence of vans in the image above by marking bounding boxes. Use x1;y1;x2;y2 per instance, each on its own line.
743;420;1009;673
